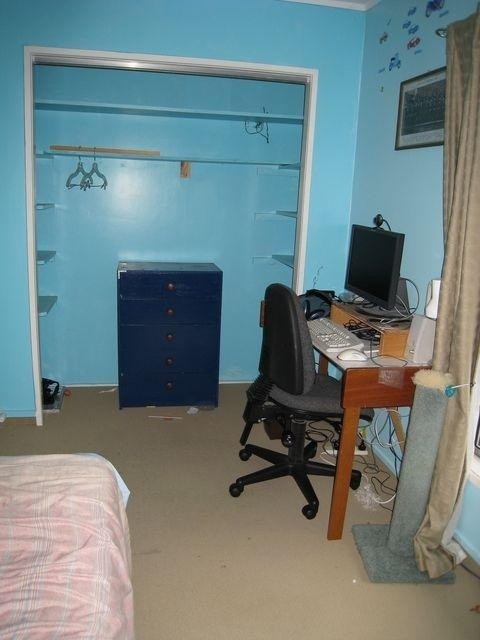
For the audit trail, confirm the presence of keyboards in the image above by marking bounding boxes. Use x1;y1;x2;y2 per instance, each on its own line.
305;317;364;353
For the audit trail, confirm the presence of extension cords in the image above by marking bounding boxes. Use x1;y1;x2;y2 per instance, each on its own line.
324;444;369;456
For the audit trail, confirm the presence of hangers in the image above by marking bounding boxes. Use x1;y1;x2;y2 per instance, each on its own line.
80;147;109;192
66;146;94;188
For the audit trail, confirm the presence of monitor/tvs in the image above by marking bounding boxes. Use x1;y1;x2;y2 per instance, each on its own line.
344;224;405;317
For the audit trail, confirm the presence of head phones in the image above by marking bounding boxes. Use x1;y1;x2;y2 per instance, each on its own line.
302;289;332;320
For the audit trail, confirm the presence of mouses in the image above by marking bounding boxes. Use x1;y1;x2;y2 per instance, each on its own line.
337;348;367;361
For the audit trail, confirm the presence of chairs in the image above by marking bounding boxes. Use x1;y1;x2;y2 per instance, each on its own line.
228;282;375;520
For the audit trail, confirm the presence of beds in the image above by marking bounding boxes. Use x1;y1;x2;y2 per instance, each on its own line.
0;453;136;640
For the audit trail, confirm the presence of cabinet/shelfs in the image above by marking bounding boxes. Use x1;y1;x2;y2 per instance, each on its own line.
253;163;301;269
114;261;223;410
35;96;304;167
32;149;68;416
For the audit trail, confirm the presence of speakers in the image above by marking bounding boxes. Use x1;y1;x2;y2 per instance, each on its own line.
404;315;436;365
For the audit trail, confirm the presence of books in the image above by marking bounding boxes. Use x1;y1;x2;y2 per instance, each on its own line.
43;385;65;414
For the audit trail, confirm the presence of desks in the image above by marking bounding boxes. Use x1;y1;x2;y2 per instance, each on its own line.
300;297;437;540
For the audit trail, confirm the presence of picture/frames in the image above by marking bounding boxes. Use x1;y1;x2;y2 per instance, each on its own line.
394;66;447;151
